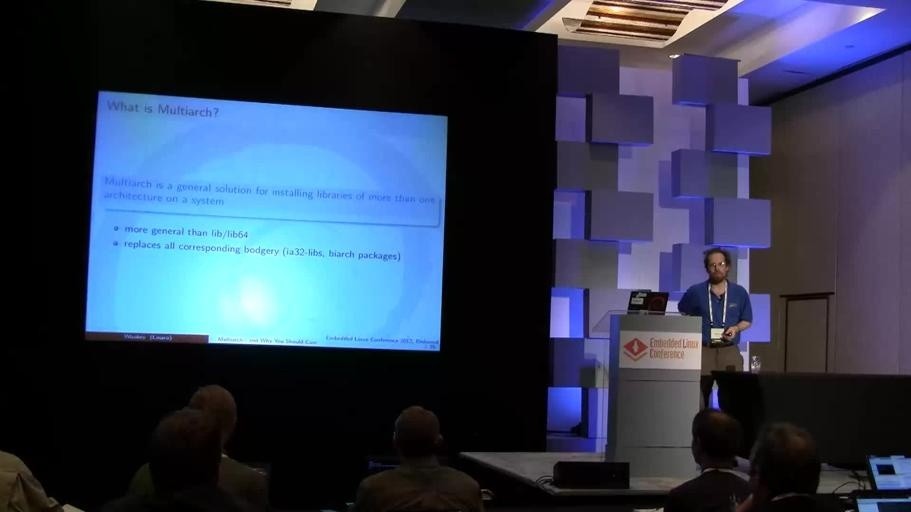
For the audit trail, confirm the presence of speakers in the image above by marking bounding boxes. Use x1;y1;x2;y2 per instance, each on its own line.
552;461;629;489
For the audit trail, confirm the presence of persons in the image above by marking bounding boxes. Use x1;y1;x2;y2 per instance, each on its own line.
0;448;67;512
127;386;270;512
679;248;755;409
105;406;256;509
735;418;855;510
663;406;751;510
352;405;485;511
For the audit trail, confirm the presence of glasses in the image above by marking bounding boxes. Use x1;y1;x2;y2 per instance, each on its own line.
709;261;726;267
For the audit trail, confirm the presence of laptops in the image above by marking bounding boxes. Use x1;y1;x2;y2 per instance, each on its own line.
627;289;669;315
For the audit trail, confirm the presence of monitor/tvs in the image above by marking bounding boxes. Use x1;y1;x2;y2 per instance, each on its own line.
865;453;911;490
851;490;911;512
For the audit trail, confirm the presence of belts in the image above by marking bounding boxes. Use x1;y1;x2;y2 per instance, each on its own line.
702;341;736;348
